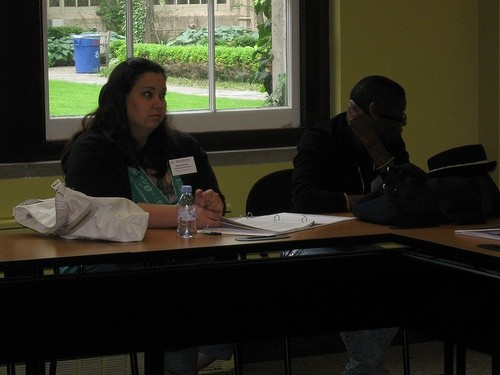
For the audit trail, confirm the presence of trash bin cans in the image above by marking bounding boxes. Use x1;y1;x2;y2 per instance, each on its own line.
72;34;101;73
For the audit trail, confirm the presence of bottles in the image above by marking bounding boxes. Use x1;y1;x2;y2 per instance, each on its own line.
177;185;199;239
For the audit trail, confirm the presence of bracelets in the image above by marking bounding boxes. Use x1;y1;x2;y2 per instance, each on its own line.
344;193;352;212
376;156;396;172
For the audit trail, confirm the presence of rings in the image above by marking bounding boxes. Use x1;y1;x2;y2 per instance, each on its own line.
207;224;209;228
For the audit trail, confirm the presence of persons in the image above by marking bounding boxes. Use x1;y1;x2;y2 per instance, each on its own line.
60;56;235;375
291;75;427;375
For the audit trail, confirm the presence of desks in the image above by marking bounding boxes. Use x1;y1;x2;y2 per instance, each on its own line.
0;213;500;375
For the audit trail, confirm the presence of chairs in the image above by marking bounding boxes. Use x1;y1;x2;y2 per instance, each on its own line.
100;30;110;66
247;169;411;375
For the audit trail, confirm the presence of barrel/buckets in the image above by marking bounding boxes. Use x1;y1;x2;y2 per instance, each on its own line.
73;36;100;73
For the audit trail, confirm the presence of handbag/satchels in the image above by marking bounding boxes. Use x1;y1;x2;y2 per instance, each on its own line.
11;179;152;243
351;144;500;228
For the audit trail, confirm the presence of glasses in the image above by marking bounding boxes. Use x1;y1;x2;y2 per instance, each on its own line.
376;112;406;124
